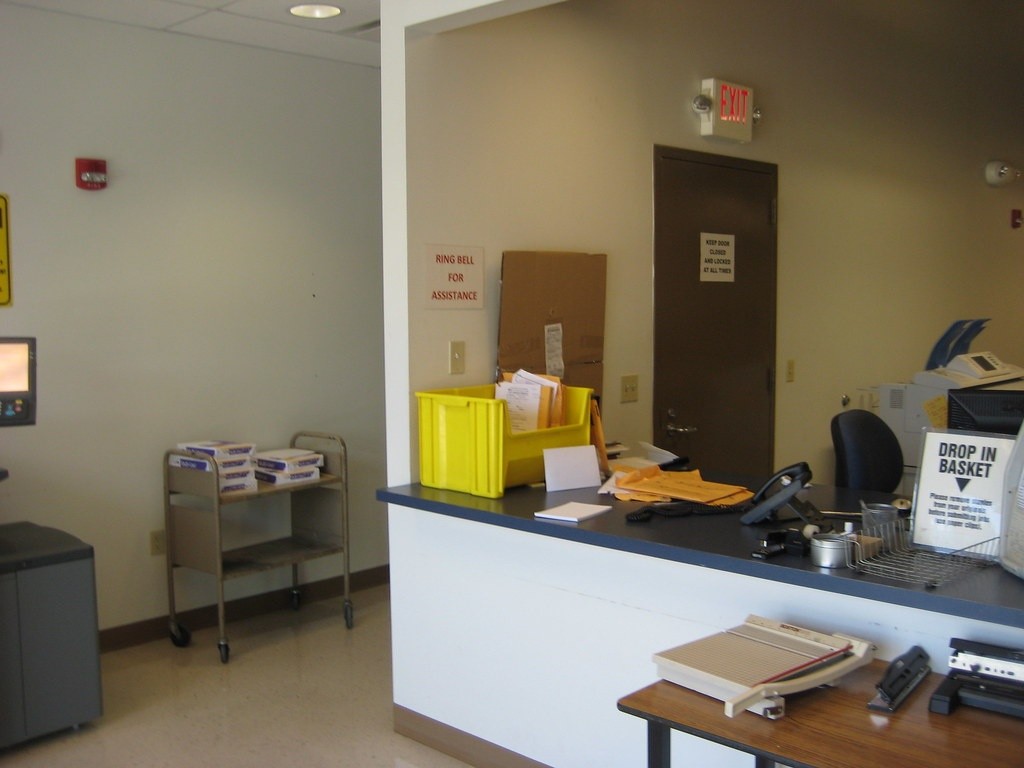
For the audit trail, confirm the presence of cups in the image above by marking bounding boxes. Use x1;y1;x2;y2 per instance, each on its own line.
862;504;897;551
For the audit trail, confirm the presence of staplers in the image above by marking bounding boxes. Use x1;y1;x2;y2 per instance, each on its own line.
751;528;801;561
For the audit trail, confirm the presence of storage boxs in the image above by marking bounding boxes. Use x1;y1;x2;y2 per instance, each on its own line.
415;384;595;499
843;515;1001;586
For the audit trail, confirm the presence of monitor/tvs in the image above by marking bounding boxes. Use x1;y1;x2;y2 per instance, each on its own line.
948;388;1024;435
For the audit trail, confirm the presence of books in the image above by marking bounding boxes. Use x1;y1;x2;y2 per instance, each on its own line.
534;501;613;522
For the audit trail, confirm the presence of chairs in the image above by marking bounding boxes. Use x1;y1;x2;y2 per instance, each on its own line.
830;409;905;492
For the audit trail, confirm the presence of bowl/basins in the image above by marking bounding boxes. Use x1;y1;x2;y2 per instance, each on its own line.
810;534;853;568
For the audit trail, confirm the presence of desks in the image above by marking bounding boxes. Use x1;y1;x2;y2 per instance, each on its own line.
617;658;1023;768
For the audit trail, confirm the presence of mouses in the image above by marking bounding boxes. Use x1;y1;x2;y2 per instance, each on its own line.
892;499;912;514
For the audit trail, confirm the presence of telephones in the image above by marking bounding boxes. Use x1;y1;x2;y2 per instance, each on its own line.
739;461;813;526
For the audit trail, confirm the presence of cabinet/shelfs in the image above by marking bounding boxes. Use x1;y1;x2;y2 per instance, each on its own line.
376;466;1023;768
163;431;354;661
